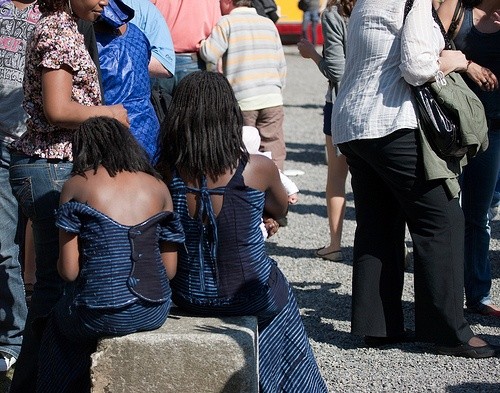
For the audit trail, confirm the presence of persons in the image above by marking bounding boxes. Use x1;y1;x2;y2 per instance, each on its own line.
9;0;129;283
332;0;500;359
298;0;360;262
253;0;278;24
149;0;223;95
119;0;176;122
201;0;287;173
0;0;43;371
9;115;186;393
239;126;299;241
436;0;500;316
152;70;328;393
93;0;159;161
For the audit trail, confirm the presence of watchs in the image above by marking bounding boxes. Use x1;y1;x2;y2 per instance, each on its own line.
298;0;321;47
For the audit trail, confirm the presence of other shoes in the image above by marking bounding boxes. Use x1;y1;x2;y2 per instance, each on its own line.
364;328;413;346
436;339;500;358
475;304;500;318
312;245;343;261
0;350;17;372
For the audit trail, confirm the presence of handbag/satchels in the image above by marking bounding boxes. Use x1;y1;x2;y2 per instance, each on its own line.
403;1;487;158
298;1;310;13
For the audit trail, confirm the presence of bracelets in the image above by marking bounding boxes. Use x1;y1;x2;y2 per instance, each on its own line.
466;59;473;72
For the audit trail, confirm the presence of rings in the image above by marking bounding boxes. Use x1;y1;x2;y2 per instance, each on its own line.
483;80;488;85
